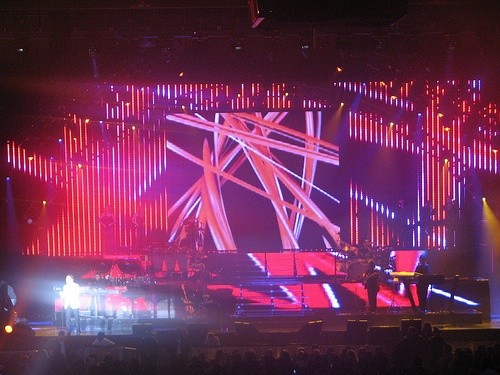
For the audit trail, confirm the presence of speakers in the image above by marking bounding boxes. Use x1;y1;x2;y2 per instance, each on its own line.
347;320;367;333
132;323;153;336
401;318;422;335
235;322;257;335
187;322;209;337
370;326;399;337
298;321;322;334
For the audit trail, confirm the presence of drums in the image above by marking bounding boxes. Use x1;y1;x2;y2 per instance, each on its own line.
345;259;364;279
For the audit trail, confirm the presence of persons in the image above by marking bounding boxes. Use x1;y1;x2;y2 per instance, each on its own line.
363;258;380;314
358;236;373;254
0;321;500;375
414;255;431;311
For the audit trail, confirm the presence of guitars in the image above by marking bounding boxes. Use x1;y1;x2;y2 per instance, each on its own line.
181;285;195;315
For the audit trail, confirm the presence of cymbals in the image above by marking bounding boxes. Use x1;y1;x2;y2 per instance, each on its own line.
339;246;356;253
359;242;375;245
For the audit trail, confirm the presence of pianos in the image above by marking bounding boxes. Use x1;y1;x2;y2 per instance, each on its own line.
391;273;446;312
